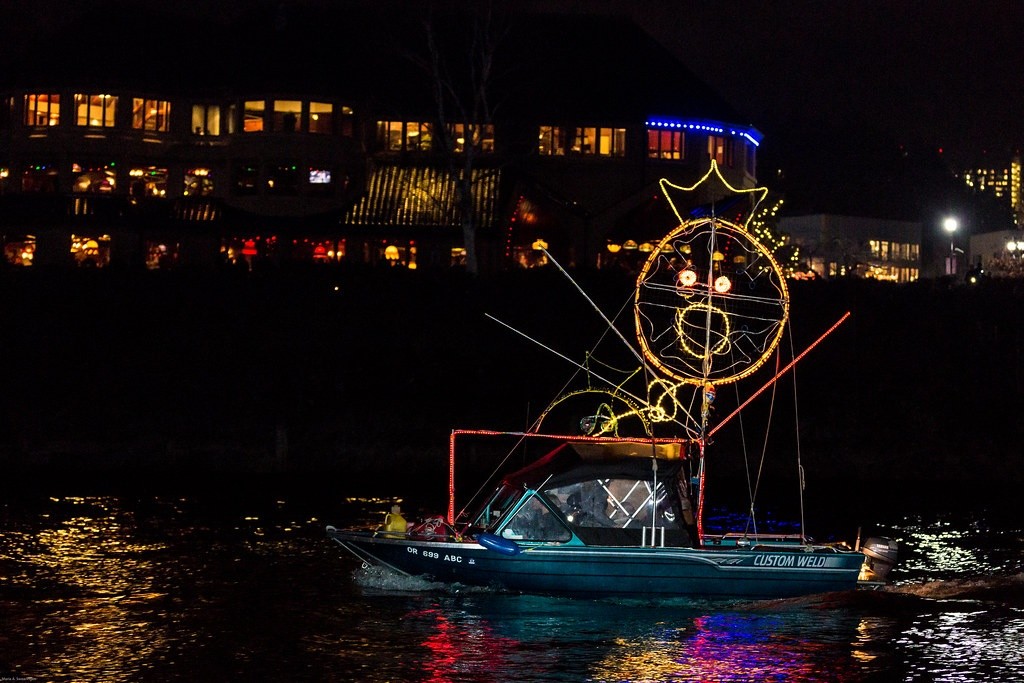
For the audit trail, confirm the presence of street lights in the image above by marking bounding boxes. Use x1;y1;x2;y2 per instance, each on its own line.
941;216;963;270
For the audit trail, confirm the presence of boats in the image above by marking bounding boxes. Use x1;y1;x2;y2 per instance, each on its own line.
319;163;903;594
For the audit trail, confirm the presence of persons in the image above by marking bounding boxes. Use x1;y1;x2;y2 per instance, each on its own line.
383;504;408;540
